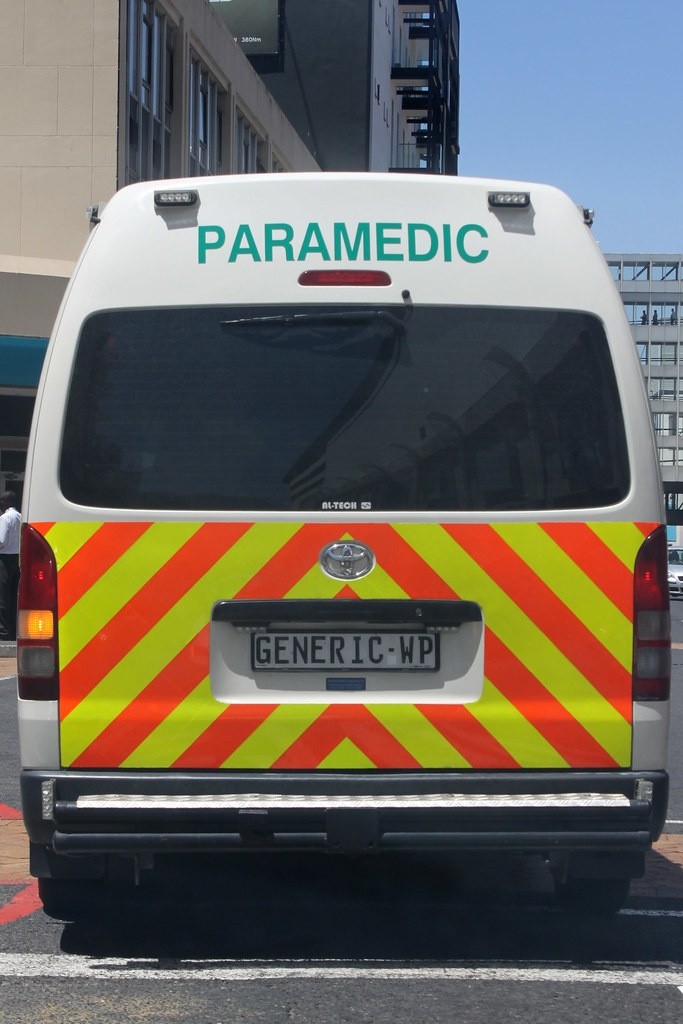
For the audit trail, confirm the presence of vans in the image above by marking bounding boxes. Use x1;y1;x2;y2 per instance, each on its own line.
11;165;673;921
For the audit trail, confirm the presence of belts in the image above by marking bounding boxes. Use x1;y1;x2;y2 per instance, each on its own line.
0;554;19;558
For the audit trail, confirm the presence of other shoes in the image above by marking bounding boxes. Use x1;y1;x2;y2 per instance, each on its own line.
0;633;16;640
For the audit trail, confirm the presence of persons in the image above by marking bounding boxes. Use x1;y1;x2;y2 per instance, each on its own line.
0;490;23;640
